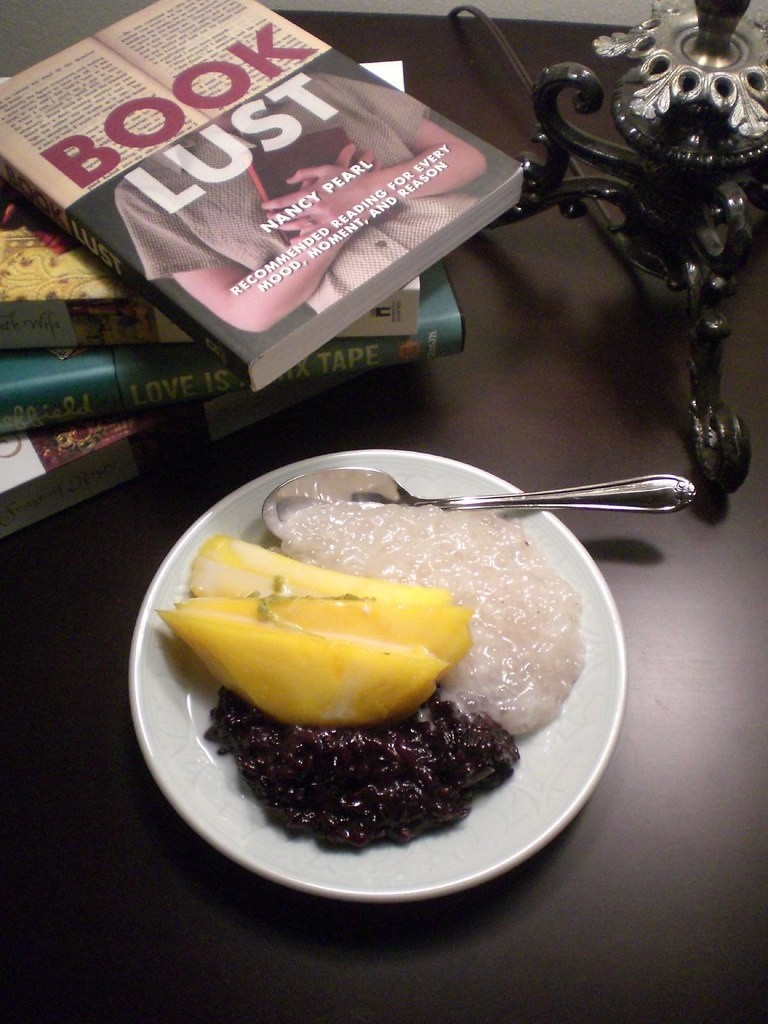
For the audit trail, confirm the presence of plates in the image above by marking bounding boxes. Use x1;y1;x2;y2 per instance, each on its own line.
126;447;627;905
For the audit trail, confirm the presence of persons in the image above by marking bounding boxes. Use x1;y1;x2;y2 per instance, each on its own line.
114;72;486;333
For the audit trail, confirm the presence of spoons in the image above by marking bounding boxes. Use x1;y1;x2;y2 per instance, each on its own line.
261;466;696;545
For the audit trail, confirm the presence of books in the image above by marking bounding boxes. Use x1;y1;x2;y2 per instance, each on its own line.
1;0;527;545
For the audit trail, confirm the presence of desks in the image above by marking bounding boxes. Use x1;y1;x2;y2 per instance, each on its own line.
0;12;768;1024
